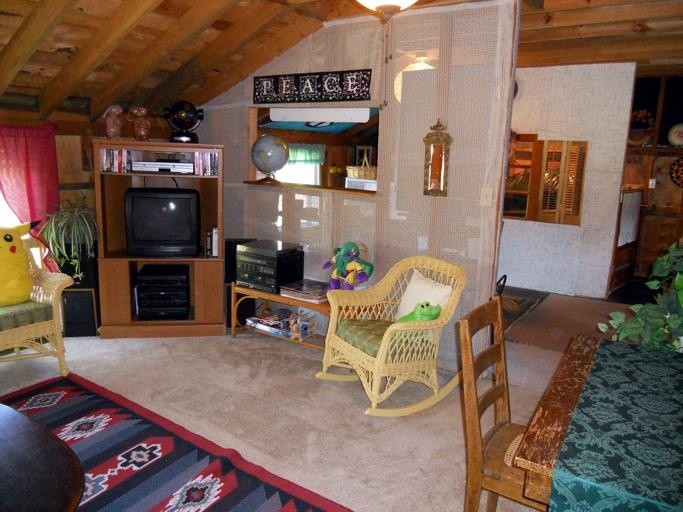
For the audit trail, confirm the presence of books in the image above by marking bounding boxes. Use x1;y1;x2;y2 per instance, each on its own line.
100;149;141;175
282;278;328;305
193;152;219;176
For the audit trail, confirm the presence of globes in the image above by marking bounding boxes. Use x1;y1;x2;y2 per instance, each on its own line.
250;135;289;184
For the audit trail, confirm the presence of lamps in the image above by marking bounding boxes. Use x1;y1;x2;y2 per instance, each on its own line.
356;0;419;21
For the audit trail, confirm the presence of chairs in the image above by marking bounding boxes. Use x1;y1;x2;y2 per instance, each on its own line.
316;255;469;418
1;239;75;377
457;296;551;512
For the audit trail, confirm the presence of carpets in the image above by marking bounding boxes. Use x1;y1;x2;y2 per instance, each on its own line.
0;372;353;511
495;285;549;335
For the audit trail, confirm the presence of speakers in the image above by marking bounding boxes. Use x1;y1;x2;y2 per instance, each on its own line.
59;241;101;336
225;238;257;328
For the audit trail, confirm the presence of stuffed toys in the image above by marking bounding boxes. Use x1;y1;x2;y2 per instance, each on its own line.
1;219;43;307
321;243;372;290
399;301;441;326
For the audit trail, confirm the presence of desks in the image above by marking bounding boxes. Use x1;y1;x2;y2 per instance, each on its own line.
0;403;84;512
511;332;682;511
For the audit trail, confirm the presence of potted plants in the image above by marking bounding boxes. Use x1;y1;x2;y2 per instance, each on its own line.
37;194;100;336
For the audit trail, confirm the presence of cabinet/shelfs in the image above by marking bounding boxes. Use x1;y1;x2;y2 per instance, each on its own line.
230;280;385;375
90;135;227;339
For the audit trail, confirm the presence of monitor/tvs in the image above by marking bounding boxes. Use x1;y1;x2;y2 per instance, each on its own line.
124;187;200;256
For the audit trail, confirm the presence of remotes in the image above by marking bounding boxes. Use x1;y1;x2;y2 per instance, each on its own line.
156;159;180;162
